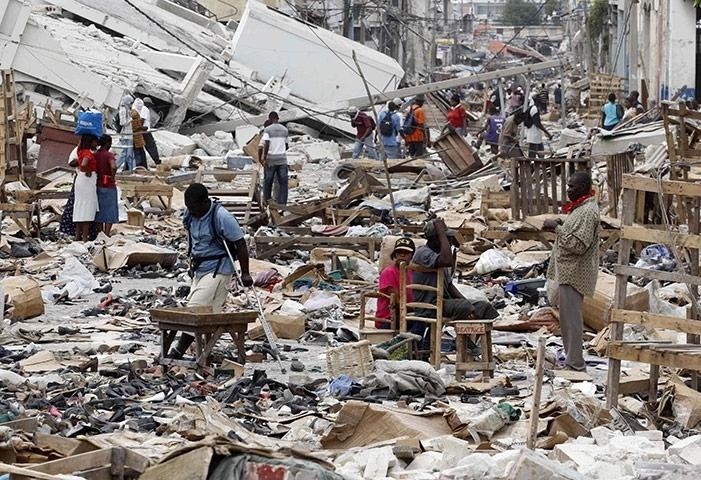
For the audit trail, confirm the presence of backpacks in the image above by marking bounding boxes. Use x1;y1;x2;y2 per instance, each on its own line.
401;109;419;135
111;112;122;133
380;110;397;137
523;105;535;129
367;115;377;130
615;103;624;120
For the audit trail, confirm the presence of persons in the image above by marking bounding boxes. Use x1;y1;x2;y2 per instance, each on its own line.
601;90;646;130
94;133;120;238
258;120;290;203
140;96;163;166
168;182;254;358
131;98;150;171
447;84;562;157
348;97;429;157
374;238;433;365
410;215;499;356
113;94;134;174
262;110;290;208
74;131;101;246
60;134;103;241
543;169;603;373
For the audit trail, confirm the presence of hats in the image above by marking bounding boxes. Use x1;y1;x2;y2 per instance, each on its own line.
142;96;155;105
423;218;458;238
392;97;404;105
415;93;426;101
393;237;416;255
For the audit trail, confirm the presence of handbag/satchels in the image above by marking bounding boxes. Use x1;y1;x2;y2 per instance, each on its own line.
242;133;264;163
74;106;106;138
